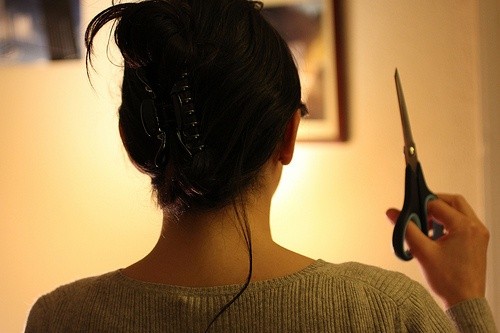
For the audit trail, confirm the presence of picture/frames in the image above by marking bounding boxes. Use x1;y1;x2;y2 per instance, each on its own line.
256;0;346;143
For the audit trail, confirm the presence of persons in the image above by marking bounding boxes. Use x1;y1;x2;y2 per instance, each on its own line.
24;0;499;332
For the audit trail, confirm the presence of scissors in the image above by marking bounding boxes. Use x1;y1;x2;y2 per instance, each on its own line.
393;68;444;261
